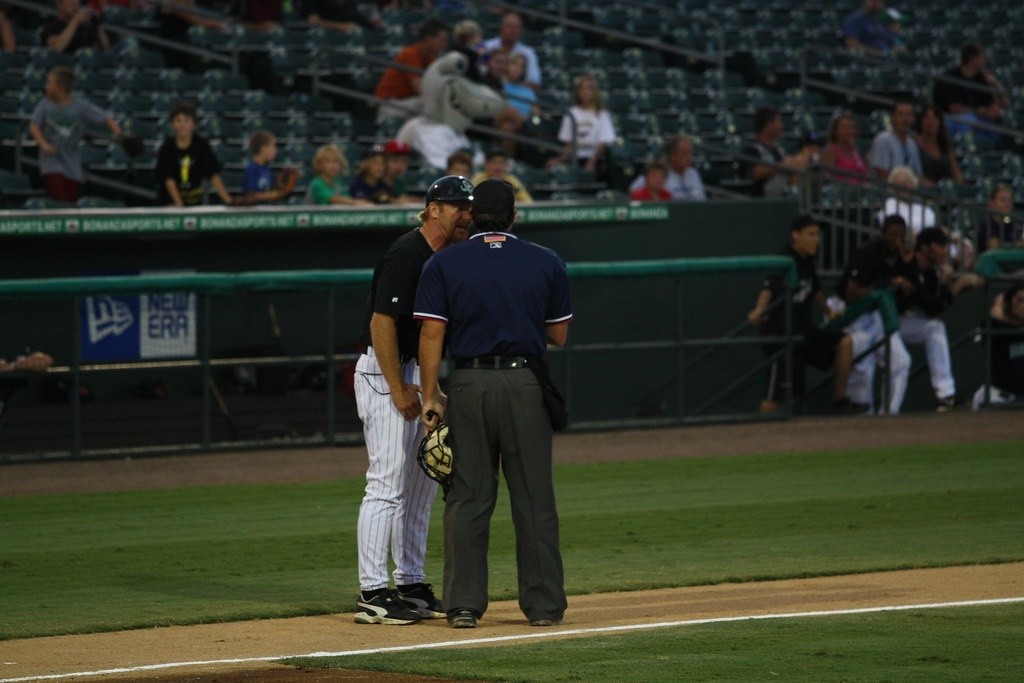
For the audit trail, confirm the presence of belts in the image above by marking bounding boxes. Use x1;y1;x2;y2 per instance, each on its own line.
453;357;529;369
364;346;410;363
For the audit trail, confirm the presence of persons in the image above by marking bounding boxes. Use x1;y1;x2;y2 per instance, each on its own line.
41;0;108;53
88;1;476;37
30;68;121;209
844;0;908;58
737;101;962;199
239;131;534;206
932;45;1010;144
155;107;233;210
0;11;15;96
412;177;573;629
377;12;707;205
354;174;475;625
747;166;1023;418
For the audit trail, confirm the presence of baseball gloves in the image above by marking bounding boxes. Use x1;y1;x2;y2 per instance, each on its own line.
416;420;456;485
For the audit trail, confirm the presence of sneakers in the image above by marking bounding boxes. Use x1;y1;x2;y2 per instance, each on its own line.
532;612;564;626
353;589;420;626
394;583;447;618
448;610;476;627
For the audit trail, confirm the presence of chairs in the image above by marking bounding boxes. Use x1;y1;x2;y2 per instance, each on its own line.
0;0;1024;245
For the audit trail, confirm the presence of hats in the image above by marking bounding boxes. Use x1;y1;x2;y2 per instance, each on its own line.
384;142;411;153
360;144;384;159
425;176;474;201
473;178;515;213
915;227;958;246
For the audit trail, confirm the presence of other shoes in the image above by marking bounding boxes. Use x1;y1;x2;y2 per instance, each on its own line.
937;398;956;411
973;385;1010;408
